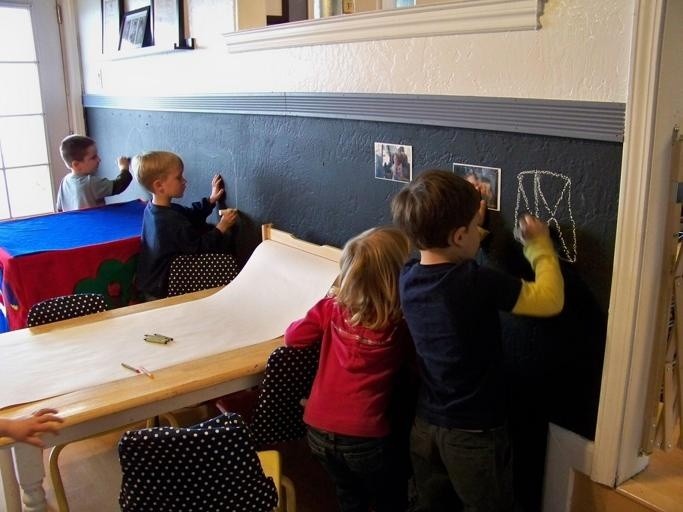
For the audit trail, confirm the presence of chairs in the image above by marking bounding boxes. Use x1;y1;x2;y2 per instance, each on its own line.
27;294;109;329
256;348;321;448
167;252;238;297
116;413;298;512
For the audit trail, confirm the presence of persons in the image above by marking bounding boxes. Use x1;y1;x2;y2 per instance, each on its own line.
128;150;237;301
56;135;132;212
0;408;65;447
375;145;411;181
391;171;566;510
283;226;418;509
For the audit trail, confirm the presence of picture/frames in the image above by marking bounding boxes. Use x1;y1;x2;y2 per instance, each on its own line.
117;5;151;49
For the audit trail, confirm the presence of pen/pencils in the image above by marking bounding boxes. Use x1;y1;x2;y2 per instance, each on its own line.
140;367;152;377
121;363;140;373
144;333;173;344
219;208;237;216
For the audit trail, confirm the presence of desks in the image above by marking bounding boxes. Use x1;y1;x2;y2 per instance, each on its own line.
1;199;151;333
1;226;348;512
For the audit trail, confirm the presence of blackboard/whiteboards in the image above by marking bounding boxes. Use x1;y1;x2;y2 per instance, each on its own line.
84;106;624;443
